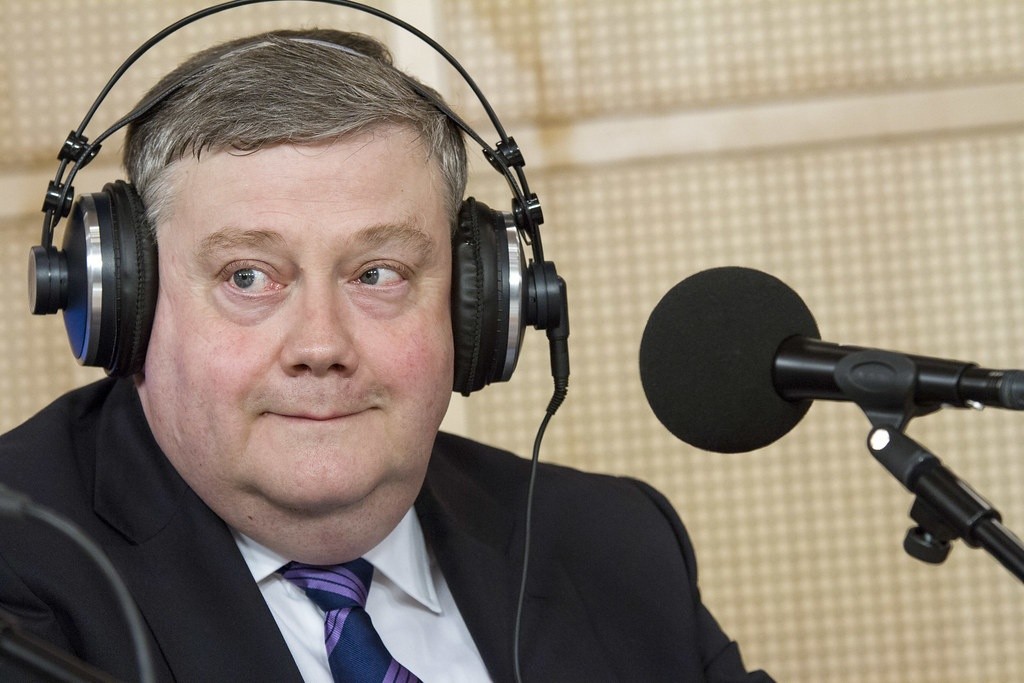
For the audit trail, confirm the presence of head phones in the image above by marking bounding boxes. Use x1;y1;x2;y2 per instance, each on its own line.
26;0;568;411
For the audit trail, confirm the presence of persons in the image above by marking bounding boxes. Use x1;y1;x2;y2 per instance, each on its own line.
0;30;772;683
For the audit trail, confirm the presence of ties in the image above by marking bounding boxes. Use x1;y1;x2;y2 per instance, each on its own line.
279;559;425;683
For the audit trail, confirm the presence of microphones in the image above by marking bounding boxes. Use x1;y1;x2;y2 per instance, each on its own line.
639;265;1022;454
